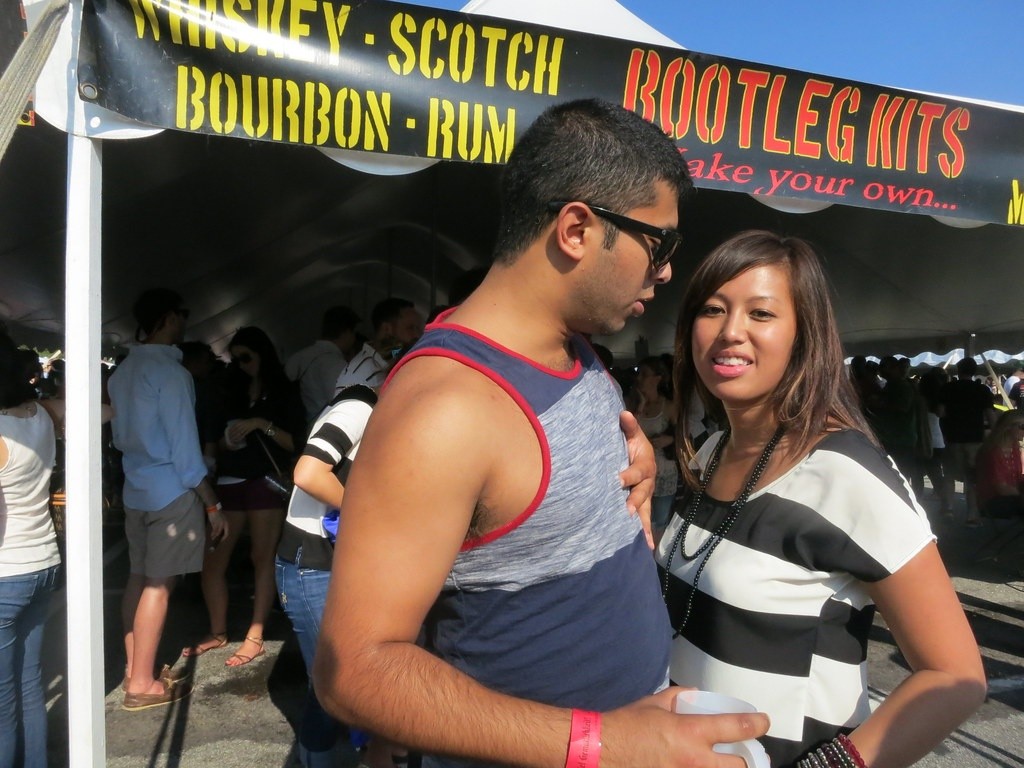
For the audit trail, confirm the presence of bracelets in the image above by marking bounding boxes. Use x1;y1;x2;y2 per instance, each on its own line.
789;732;867;768
264;420;281;440
565;706;603;768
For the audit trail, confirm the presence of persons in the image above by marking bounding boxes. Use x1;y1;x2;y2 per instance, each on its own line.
0;284;1024;768
311;98;771;768
653;227;988;768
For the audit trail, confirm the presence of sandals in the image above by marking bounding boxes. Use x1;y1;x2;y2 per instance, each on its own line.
182;630;229;658
226;634;267;667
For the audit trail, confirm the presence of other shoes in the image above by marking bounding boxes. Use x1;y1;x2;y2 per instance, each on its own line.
945;509;955;524
966;519;983;528
122;669;190;693
122;676;192;710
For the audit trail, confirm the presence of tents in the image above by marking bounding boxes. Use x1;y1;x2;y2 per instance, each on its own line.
0;0;1023;370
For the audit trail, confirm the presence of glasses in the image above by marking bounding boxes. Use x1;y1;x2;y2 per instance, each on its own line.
1013;422;1024;429
170;309;191;319
231;353;259;364
548;198;684;274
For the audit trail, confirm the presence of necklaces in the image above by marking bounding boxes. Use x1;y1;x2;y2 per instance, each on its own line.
663;420;787;641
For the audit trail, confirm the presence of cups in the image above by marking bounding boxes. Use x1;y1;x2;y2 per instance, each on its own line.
673;690;770;768
